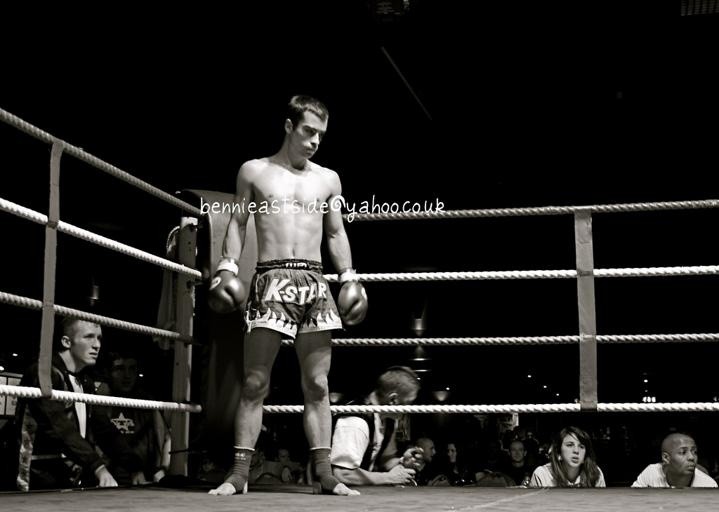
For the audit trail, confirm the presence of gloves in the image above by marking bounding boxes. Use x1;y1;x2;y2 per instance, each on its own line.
337;268;368;325
208;257;247;313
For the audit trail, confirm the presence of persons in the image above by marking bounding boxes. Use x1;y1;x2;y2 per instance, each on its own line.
208;89;370;496
0;293;719;490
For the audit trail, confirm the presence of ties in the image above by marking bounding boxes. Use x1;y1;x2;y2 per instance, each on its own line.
375;418;394;463
